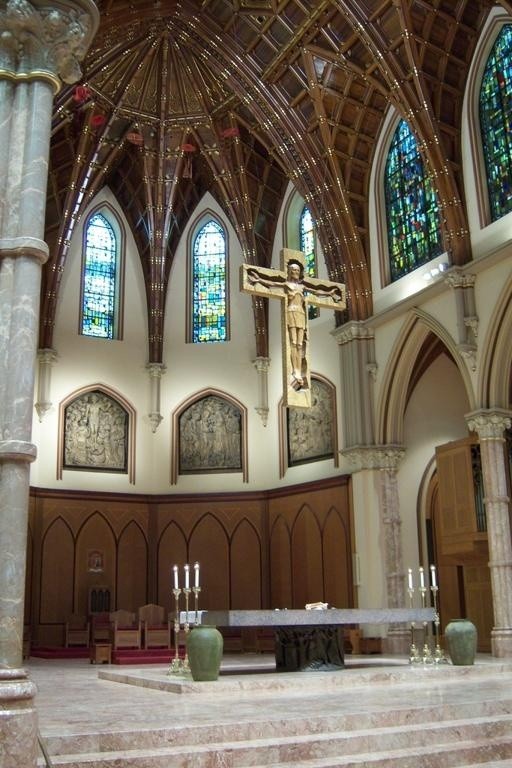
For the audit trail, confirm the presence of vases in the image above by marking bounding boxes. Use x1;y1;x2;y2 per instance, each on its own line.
187;623;223;681
445;619;478;664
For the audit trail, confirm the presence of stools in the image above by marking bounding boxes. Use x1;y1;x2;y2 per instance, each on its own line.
90;644;112;664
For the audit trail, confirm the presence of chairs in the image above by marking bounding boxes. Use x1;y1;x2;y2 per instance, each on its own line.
64;582;278;654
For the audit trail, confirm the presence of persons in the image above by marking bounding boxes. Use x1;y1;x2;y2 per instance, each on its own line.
290;392;333;456
245;261;344;391
65;393;126;469
178;401;242;468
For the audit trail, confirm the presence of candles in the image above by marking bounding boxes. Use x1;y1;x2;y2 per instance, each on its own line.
173;561;200;588
408;565;437;589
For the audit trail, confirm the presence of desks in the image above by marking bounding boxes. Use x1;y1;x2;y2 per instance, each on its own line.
181;608;435;671
